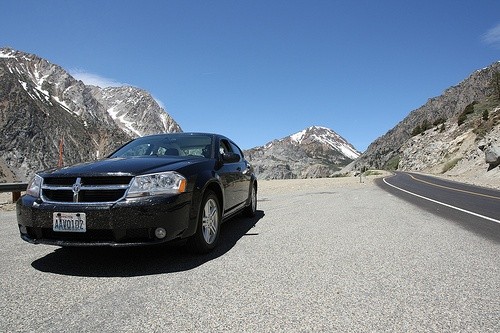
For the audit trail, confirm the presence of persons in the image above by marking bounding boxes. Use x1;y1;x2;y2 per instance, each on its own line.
202;144;211;158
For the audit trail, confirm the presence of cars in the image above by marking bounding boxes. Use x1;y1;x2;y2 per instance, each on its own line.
16;133;258;254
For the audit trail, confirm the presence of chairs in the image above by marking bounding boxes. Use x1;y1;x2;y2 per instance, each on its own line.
164;148;179;156
